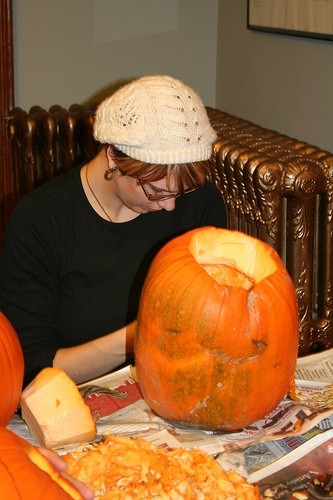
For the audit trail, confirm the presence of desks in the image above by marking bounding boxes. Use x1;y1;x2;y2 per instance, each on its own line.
8;347;333;500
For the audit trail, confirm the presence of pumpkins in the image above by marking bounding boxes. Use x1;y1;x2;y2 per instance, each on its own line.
0;311;24;432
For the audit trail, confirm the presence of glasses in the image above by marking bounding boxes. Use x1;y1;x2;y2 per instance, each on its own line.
136;176;197;202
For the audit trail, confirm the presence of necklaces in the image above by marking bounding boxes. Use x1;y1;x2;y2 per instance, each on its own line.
85;163;112;222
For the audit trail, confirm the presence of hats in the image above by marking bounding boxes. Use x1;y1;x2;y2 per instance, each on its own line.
93;75;217;164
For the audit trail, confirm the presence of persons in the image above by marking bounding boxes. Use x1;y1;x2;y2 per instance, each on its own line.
1;75;227;385
